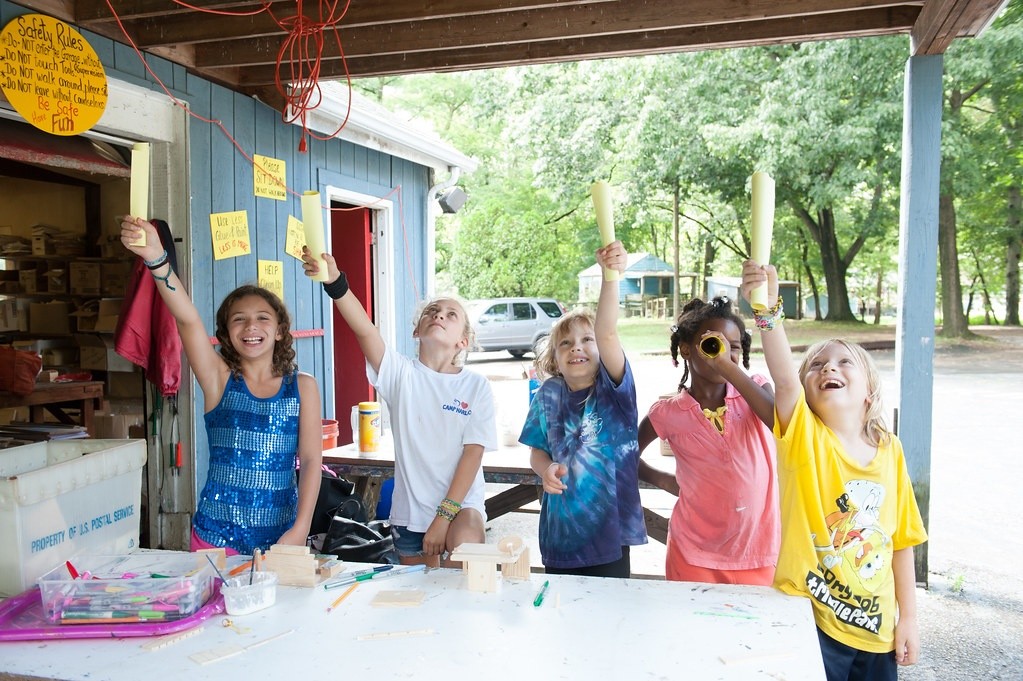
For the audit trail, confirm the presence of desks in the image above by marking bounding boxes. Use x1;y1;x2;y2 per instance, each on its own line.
322;442;546;524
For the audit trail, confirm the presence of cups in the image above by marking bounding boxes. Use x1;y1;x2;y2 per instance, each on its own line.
358;401;382;452
350;405;359;444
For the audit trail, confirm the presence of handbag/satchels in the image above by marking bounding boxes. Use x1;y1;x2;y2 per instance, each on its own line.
0;345;41;396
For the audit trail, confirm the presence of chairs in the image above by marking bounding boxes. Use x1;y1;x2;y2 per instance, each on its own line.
627;293;673;319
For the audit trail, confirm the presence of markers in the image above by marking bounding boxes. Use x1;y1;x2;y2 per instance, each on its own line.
47;561;208;623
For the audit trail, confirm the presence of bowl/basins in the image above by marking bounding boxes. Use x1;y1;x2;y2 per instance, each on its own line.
219;571;278;616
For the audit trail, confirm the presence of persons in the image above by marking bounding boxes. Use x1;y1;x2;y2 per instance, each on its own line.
301;244;498;568
121;215;322;553
639;296;781;585
519;241;648;580
742;258;929;681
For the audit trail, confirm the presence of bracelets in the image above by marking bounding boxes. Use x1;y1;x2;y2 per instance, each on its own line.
143;250;176;291
323;271;349;299
436;498;462;522
753;298;786;332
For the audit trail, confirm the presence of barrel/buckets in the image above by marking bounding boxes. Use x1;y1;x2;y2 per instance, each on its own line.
321;419;339;450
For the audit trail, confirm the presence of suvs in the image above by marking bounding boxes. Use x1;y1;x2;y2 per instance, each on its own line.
460;296;567;358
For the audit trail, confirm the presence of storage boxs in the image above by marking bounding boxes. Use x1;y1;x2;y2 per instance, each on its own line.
0;231;144;439
35;552;220;622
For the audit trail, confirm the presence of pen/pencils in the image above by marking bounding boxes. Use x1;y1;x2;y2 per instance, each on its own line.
327;582;359;612
372;565;426;579
337;566;393;579
533;580;549;607
324;571;380;589
230;555;266;576
249;555;256;584
206;554;229;587
